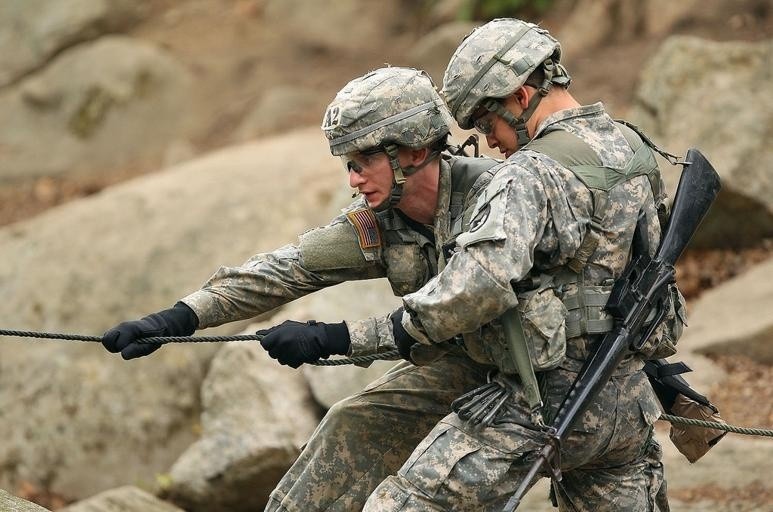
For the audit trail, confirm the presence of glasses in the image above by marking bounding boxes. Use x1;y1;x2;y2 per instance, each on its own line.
471;98;503;134
338;146;385;177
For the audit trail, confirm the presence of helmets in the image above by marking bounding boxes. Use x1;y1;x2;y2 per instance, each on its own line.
322;64;455;156
438;16;562;131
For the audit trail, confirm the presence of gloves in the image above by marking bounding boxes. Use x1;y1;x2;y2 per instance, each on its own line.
103;302;197;361
392;305;427;365
255;320;350;369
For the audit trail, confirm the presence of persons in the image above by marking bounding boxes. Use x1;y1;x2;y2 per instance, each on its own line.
245;13;671;511
99;61;519;512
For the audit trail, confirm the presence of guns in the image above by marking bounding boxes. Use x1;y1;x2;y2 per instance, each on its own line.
500;149;721;512
643;360;727;464
